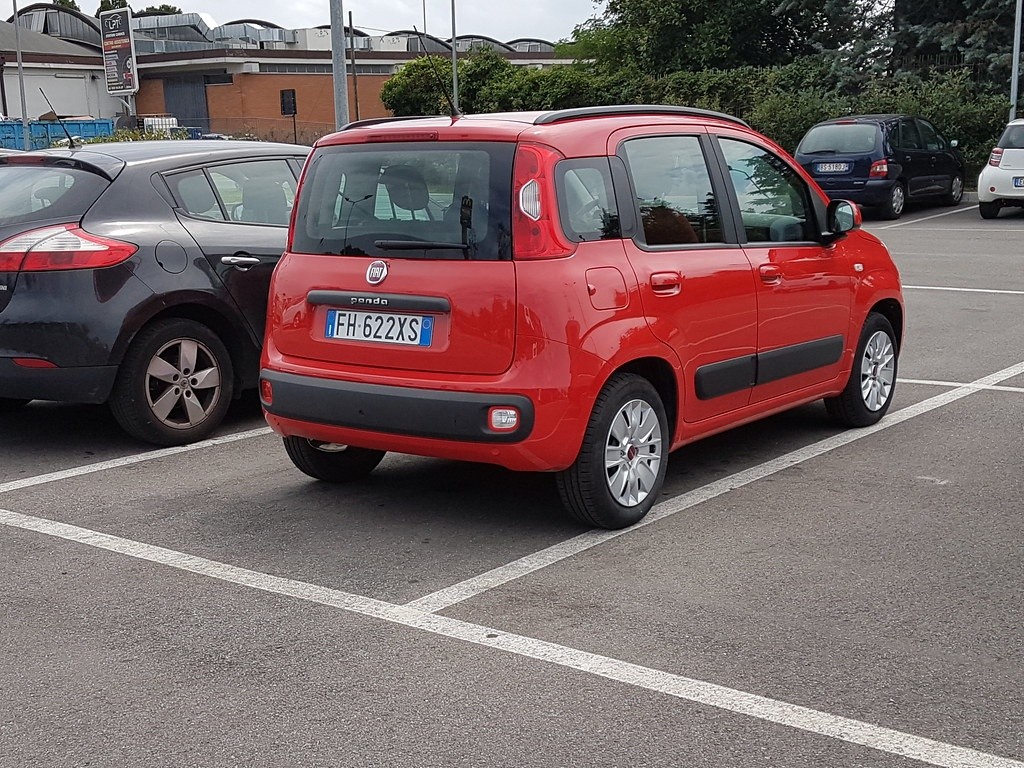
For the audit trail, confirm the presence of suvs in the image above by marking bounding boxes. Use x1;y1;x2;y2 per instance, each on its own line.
258;104;905;530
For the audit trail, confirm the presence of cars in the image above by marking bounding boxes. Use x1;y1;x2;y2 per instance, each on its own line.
0;141;444;446
794;114;965;220
977;119;1024;220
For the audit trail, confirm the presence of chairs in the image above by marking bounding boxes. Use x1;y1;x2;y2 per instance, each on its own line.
177;175;216;219
630;151;699;245
239;180;288;226
